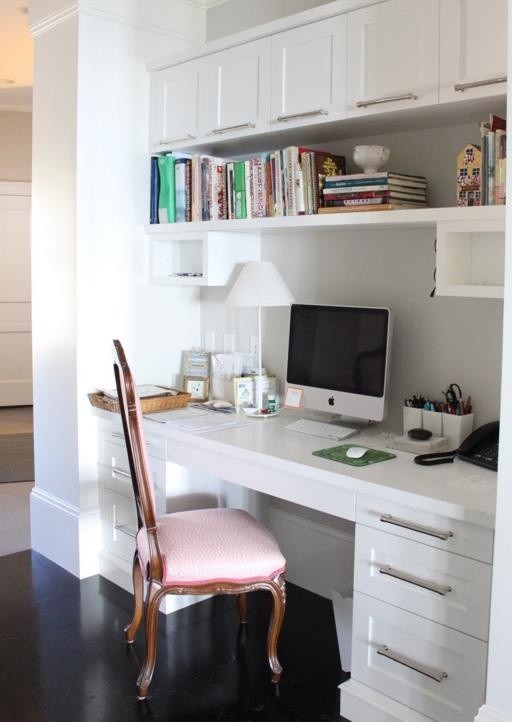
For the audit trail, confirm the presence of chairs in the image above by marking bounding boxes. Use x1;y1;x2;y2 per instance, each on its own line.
107;340;284;699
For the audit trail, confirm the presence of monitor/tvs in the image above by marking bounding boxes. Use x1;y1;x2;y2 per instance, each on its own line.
285;303;393;434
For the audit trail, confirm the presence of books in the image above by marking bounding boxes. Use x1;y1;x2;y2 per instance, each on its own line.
148;145;427;226
98;349;252;435
479;115;506;206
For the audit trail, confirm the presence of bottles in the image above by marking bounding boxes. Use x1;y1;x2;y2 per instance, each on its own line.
268;394;276;413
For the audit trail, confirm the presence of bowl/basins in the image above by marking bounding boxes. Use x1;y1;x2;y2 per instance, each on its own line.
353;144;390;174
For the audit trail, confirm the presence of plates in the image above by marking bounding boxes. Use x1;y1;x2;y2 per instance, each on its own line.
242;407;257;415
251;414;278;418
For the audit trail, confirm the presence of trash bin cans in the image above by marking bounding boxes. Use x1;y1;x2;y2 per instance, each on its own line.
331;584;354;673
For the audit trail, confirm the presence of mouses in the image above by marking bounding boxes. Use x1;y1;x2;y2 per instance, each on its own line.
346;447;369;459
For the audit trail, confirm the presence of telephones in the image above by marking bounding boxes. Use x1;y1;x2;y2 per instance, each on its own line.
457;420;500;472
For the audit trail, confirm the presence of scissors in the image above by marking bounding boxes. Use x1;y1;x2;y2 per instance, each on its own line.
446;384;462;403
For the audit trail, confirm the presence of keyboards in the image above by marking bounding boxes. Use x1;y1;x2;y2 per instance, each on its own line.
284;418;357;441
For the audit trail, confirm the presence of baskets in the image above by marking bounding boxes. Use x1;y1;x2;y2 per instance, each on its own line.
87;384;193;414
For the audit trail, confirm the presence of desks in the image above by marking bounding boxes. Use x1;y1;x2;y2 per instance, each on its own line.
104;394;500;722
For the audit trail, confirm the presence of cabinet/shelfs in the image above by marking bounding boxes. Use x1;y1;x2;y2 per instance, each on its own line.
156;203;505;301
146;1;509;153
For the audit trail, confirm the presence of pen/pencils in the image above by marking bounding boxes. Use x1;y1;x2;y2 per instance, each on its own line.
404;391;472;416
